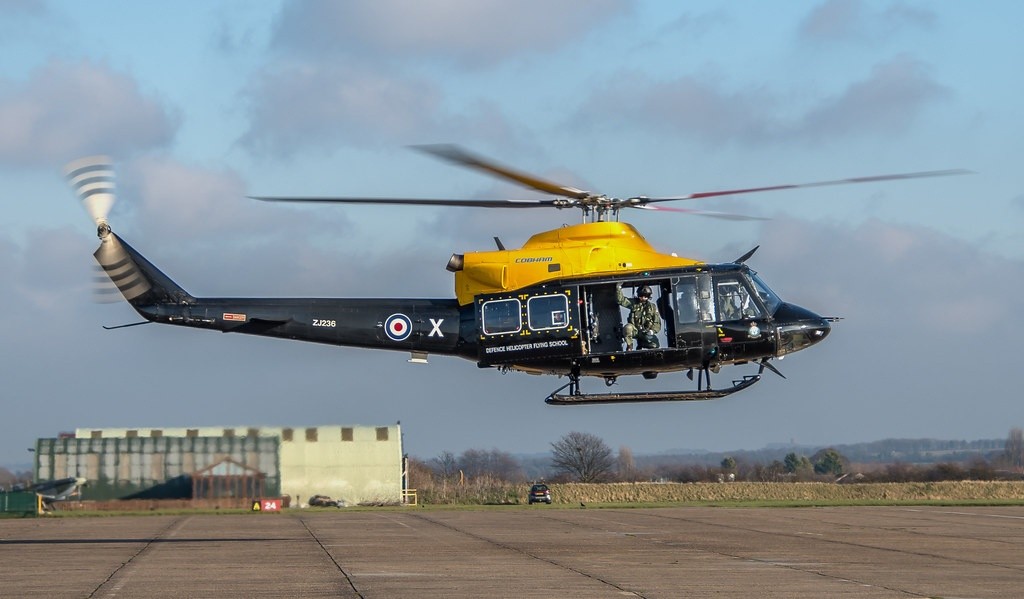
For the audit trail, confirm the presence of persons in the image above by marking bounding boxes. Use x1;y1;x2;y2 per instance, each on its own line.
718;285;754;320
616;281;663;352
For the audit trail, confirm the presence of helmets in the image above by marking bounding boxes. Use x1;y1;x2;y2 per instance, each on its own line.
637;285;652;294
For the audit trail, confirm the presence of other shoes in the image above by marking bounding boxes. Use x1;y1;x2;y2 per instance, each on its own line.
626;344;633;351
636;340;642;350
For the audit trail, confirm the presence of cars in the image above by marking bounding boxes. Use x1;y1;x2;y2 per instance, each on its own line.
528;484;552;505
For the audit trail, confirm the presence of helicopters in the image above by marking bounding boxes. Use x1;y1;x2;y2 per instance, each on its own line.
62;142;977;406
